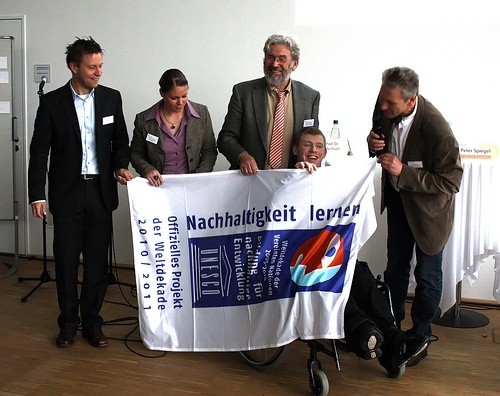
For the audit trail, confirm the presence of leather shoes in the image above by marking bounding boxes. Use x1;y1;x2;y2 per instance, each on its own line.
81;327;109;347
56;328;78;347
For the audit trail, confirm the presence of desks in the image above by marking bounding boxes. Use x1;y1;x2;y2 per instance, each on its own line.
433;144;500;328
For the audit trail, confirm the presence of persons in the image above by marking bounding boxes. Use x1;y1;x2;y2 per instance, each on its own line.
130;68;218;186
367;67;463;368
217;34;320;176
292;127;429;371
27;37;135;349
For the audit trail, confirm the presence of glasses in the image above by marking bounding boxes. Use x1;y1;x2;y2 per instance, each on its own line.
265;57;295;63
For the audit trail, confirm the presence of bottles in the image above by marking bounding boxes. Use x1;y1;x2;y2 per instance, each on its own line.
330;120;341;139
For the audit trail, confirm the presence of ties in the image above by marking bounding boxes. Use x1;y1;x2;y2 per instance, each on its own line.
269;89;286;169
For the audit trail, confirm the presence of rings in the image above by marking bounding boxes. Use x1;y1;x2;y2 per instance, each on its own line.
154;176;158;179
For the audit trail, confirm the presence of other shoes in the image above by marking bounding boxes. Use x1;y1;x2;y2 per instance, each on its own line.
357;327;382;360
391;333;429;369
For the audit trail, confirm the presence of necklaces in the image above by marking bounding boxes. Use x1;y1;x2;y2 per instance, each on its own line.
163;105;180;128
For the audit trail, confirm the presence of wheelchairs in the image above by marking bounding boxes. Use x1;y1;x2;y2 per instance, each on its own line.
239;258;406;396
